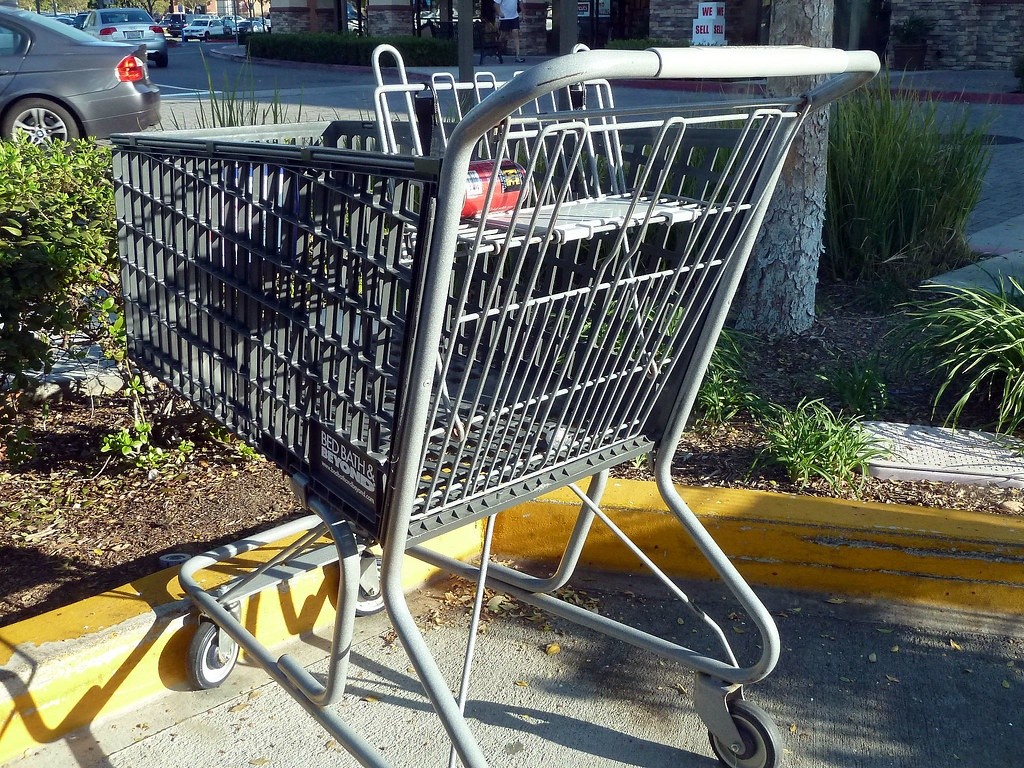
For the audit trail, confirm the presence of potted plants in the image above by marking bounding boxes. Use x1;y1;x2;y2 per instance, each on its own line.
888;11;935;72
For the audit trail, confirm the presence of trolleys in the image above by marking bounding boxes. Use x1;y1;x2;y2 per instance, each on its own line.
107;40;884;768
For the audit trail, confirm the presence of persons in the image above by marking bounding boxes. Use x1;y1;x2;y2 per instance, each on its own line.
494;0;525;62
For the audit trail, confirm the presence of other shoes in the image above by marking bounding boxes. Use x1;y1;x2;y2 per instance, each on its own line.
514;57;524;63
495;51;501;57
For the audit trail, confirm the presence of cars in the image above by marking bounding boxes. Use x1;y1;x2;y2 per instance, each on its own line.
39;11;271;42
80;8;169;69
0;0;161;163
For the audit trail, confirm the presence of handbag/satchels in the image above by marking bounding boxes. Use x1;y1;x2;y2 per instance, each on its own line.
517;0;522;13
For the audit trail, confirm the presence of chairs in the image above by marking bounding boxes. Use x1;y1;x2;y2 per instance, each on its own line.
475;19;507;65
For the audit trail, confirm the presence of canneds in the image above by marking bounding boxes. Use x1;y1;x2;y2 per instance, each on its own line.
462;158;528;217
